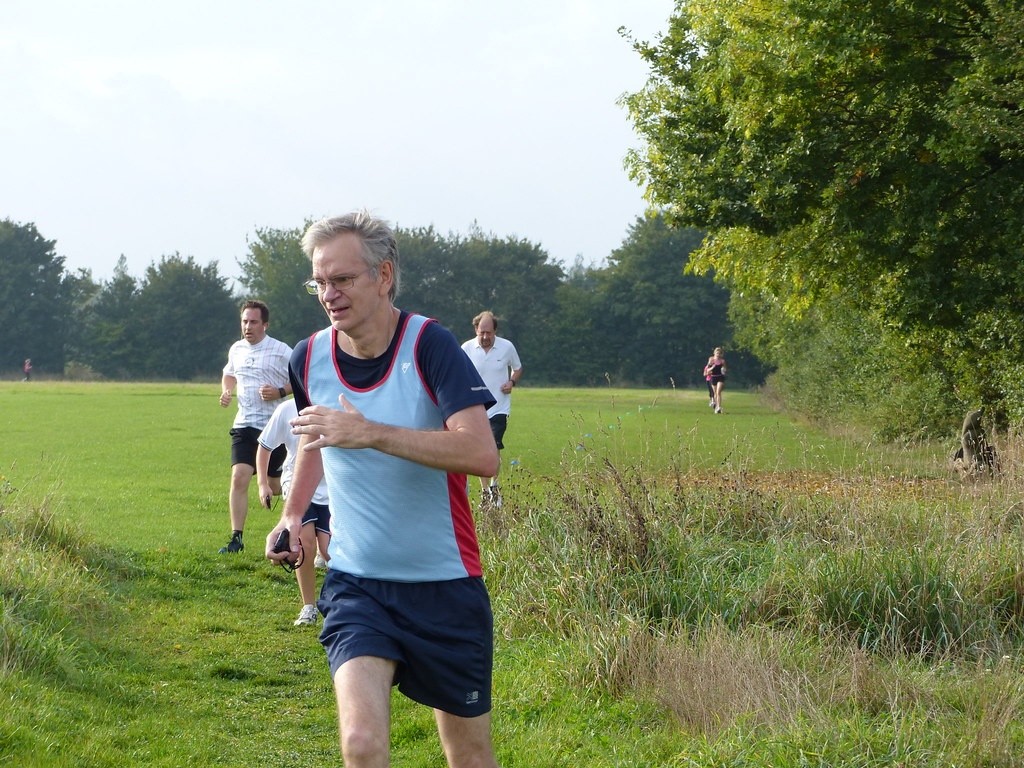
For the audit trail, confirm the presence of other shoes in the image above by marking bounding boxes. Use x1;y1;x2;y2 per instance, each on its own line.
479;491;493;509
713;402;716;409
490;485;502;509
715;407;722;413
710;402;713;406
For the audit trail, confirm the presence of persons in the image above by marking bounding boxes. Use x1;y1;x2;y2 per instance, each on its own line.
266;216;499;768
704;365;712;407
219;301;294;554
22;359;32;381
255;397;331;626
707;348;727;413
461;311;522;507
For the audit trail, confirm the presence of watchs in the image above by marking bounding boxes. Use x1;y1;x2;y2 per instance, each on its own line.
279;387;286;398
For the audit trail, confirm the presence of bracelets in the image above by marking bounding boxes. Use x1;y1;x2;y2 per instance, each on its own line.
510;379;515;387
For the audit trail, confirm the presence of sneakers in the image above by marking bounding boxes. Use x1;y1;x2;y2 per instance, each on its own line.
217;533;245;554
314;550;326;568
294;604;319;626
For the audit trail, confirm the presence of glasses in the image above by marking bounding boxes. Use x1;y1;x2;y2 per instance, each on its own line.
302;263;382;295
